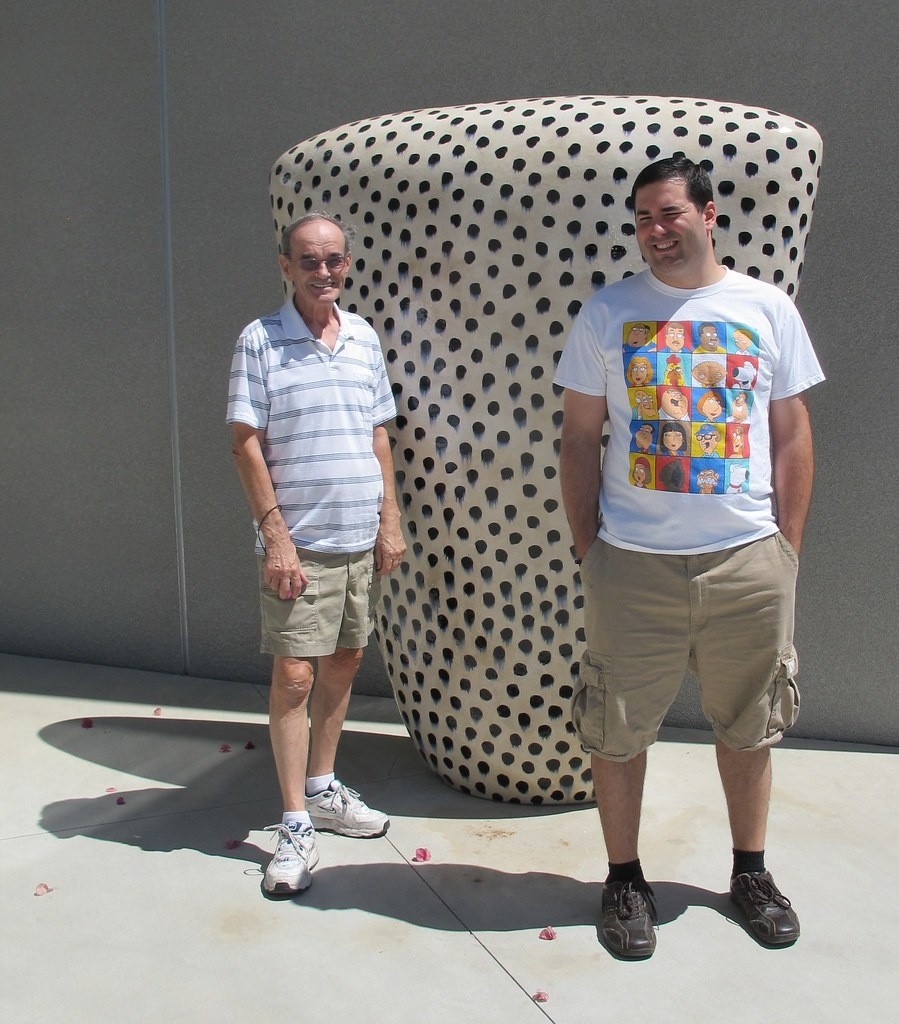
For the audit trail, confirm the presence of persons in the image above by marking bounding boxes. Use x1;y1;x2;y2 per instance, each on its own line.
558;156;816;962
227;211;410;898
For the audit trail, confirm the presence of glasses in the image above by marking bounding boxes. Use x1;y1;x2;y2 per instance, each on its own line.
286;254;347;271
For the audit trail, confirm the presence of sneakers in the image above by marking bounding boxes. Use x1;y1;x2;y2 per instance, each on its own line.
729;870;800;944
600;877;660;957
305;779;390;839
263;821;320;895
